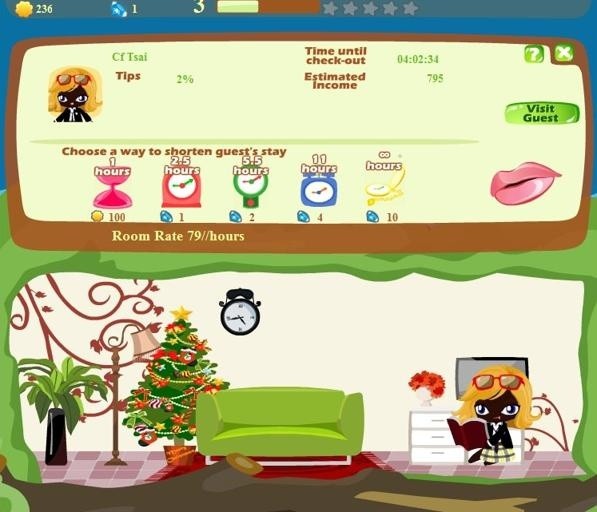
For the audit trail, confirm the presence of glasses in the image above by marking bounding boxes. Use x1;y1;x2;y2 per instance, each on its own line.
54;73;93;87
470;372;524;390
